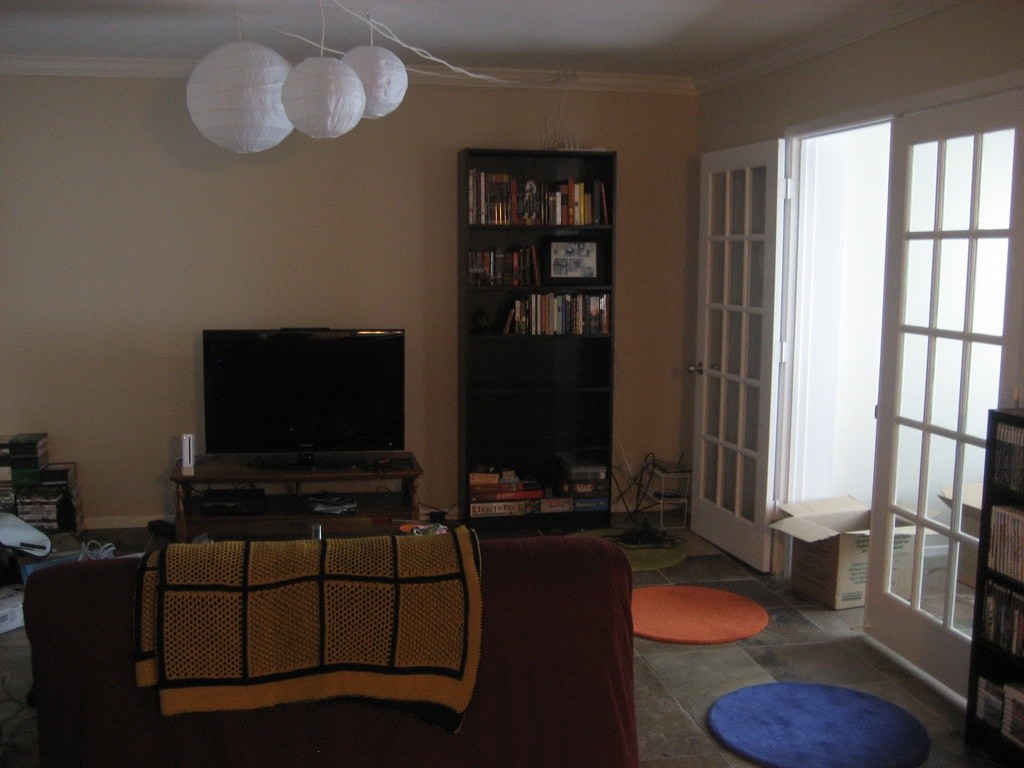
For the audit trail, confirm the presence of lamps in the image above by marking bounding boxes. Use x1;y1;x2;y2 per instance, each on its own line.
186;0;294;153
280;0;365;139
341;0;407;119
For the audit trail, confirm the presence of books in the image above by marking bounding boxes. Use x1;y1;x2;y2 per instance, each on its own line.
976;423;1024;748
310;492;357;515
0;433;83;530
468;168;608;225
471;481;543;502
503;293;610;335
468;245;540;287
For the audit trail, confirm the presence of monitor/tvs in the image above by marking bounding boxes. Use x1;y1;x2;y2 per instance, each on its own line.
199;329;407;469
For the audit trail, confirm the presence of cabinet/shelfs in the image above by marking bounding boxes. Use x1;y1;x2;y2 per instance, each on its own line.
964;408;1024;768
457;149;617;535
641;462;691;527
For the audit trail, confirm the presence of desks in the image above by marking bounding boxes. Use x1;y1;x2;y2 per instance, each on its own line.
171;453;423;537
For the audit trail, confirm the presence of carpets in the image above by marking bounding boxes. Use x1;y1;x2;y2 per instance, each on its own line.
706;682;930;768
633;585;769;645
574;529;687;572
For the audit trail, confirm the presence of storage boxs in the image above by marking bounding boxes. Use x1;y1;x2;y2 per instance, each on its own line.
938;481;983;587
769;495;944;609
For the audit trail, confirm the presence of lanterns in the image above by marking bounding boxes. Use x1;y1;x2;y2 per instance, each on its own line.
186;42;408;155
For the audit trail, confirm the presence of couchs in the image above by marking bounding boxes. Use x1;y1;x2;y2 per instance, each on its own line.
24;535;640;768
